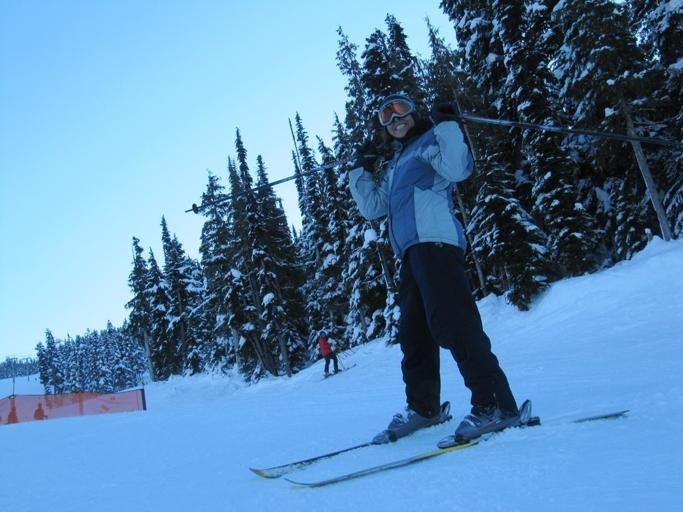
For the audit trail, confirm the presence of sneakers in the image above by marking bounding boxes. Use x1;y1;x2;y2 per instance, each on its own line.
463;403;520;438
393;407;441;438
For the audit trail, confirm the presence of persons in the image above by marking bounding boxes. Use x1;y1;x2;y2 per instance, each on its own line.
32;402;48;420
342;86;522;443
314;330;341;375
6;406;18;424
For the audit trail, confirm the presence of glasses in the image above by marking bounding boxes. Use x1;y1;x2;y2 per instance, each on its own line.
378;98;417;126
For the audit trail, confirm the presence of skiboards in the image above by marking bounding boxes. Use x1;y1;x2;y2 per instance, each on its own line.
320;364;356;380
248;411;630;487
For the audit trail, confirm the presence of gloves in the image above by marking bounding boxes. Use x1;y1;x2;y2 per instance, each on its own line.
430;97;457;127
350;140;379;173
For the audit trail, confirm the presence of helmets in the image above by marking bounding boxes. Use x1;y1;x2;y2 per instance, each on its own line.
375;94;421;135
